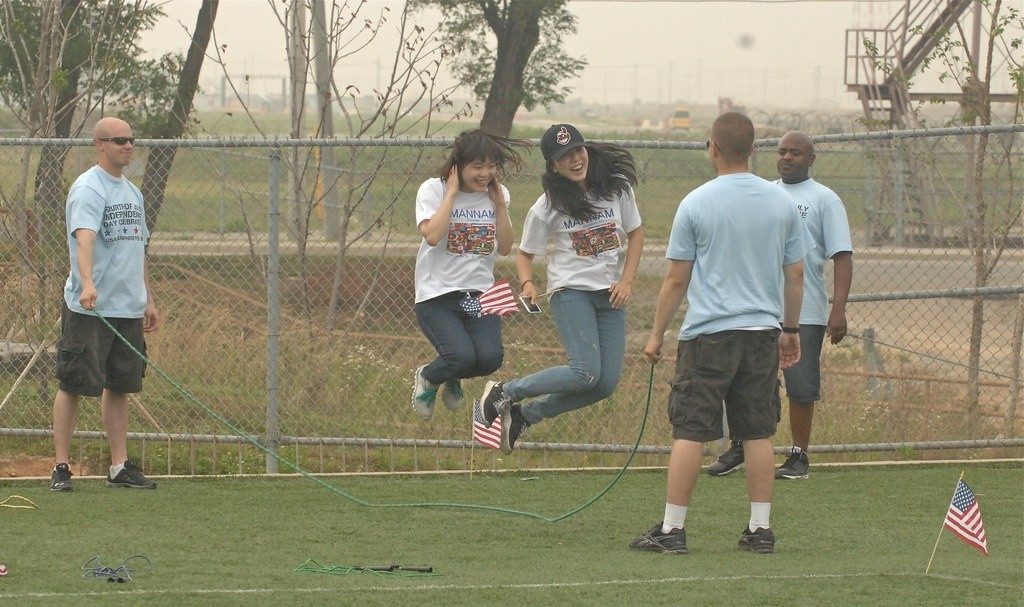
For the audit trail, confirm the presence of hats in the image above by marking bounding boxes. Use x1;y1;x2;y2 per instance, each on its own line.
541;124;591;161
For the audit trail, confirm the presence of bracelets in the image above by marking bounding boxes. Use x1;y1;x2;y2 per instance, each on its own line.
782;325;800;334
522;280;535;291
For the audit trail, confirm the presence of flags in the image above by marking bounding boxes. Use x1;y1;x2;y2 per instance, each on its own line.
942;479;987;556
472;398;504;451
459;280;519;318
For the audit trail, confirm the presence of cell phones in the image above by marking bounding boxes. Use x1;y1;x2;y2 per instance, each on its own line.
520;296;542;313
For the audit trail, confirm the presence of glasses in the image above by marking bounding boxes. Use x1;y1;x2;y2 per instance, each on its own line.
98;137;136;146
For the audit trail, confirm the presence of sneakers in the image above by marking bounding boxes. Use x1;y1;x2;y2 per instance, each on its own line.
480;379;514;429
49;462;75;491
776;446;810;479
706;440;745;476
105;460;157;489
627;519;688;554
442;379;464;411
501;402;534;455
411;363;440;420
739;524;775;553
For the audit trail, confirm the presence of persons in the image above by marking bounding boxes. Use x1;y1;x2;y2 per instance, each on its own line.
411;130;535;420
628;111;804;555
480;125;642;455
49;117;159;492
708;134;853;480
